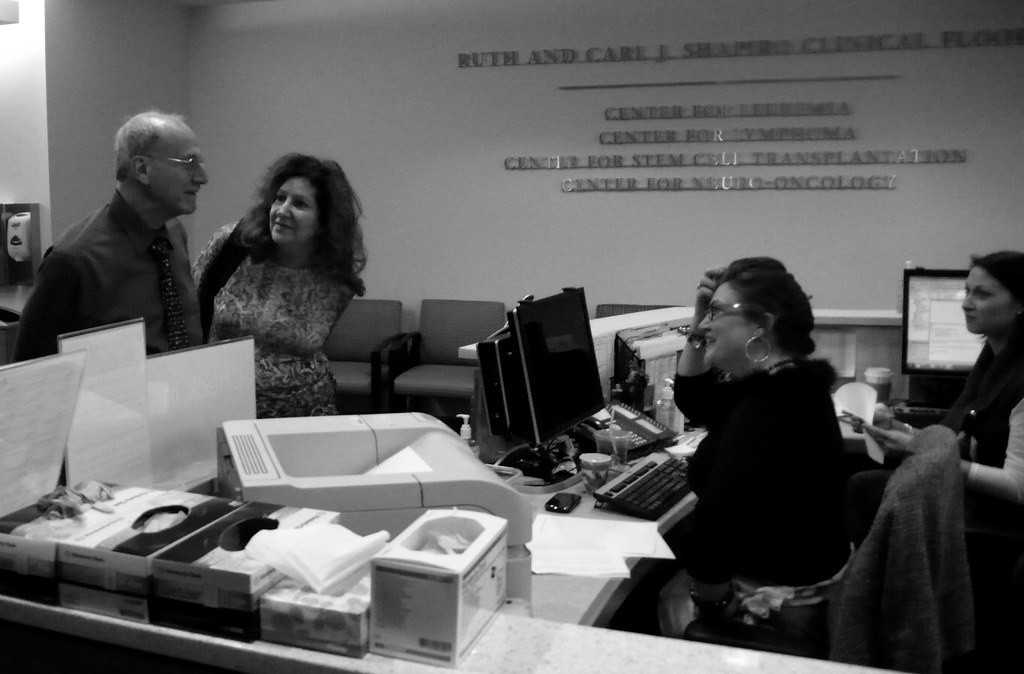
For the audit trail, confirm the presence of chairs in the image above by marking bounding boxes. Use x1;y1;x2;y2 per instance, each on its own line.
683;425;963;661
319;300;406;414
387;298;505;426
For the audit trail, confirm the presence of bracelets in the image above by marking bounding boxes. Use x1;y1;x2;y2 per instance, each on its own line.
687;331;706;348
689;579;733;611
903;423;914;435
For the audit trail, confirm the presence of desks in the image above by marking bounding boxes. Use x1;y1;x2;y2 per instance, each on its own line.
0;403;903;674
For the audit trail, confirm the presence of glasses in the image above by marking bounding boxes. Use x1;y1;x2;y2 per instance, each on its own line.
153;153;197;176
710;302;753;324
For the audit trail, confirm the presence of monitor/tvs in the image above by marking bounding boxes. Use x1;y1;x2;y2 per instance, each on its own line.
902;268;987;407
492;286;606;493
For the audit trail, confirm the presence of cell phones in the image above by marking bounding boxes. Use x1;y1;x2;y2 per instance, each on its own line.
544;492;581;514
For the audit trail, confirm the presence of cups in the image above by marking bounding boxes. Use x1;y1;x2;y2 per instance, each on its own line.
580;453;611;495
593;429;631;483
863;366;894;404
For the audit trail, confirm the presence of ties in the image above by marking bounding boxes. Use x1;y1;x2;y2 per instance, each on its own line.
150;242;187;349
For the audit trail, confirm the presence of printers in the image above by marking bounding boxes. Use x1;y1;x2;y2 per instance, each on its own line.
214;411;533;616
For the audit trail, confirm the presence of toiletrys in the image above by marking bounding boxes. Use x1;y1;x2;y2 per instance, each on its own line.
455;414;479;461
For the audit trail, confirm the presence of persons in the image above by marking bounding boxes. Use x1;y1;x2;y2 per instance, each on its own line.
604;258;854;661
851;250;1024;554
190;155;366;419
9;111;208;366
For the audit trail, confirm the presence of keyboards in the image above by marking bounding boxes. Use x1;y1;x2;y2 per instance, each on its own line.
592;452;691;522
894;405;954;430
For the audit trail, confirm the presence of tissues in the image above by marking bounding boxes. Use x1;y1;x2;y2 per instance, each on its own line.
243;521;391;661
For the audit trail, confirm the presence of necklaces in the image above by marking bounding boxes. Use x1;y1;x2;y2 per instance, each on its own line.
766;358;797;373
